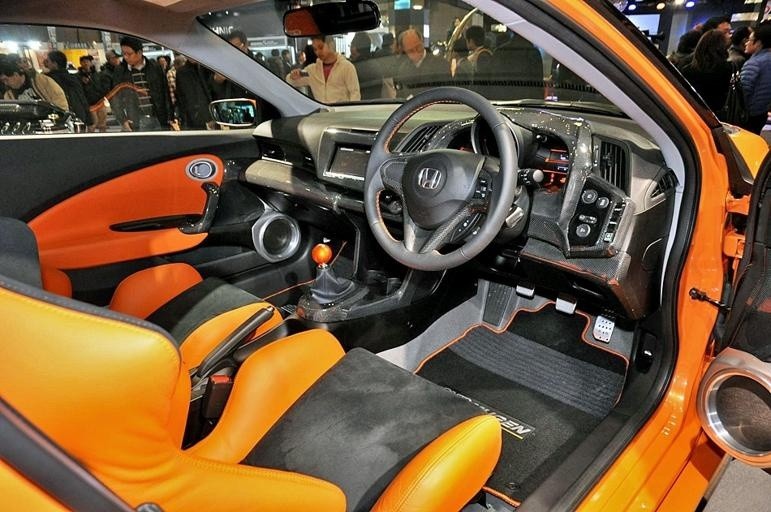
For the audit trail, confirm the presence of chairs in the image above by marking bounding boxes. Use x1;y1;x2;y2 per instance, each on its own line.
1;271;507;511
0;218;284;367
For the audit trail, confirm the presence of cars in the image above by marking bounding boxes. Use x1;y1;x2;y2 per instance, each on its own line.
0;99;87;137
0;0;771;512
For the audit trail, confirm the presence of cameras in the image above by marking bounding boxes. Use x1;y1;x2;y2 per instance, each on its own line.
299;71;309;77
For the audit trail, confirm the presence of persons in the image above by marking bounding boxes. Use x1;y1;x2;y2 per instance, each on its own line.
0;31;270;131
256;25;603;102
666;16;771;135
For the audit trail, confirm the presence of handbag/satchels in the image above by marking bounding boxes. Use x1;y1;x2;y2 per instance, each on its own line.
725;62;746;125
